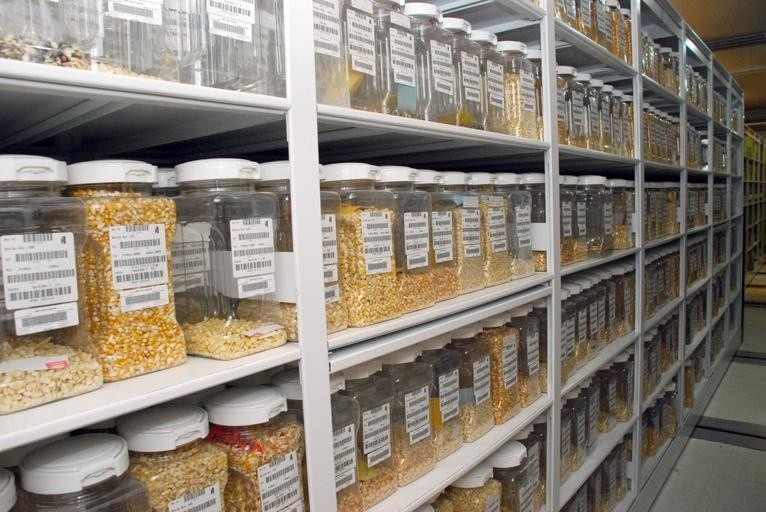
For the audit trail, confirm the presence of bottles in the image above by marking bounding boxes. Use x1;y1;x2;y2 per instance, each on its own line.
0;1;736;512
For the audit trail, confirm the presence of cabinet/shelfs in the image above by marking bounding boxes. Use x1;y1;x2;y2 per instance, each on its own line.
0;0;765;511
